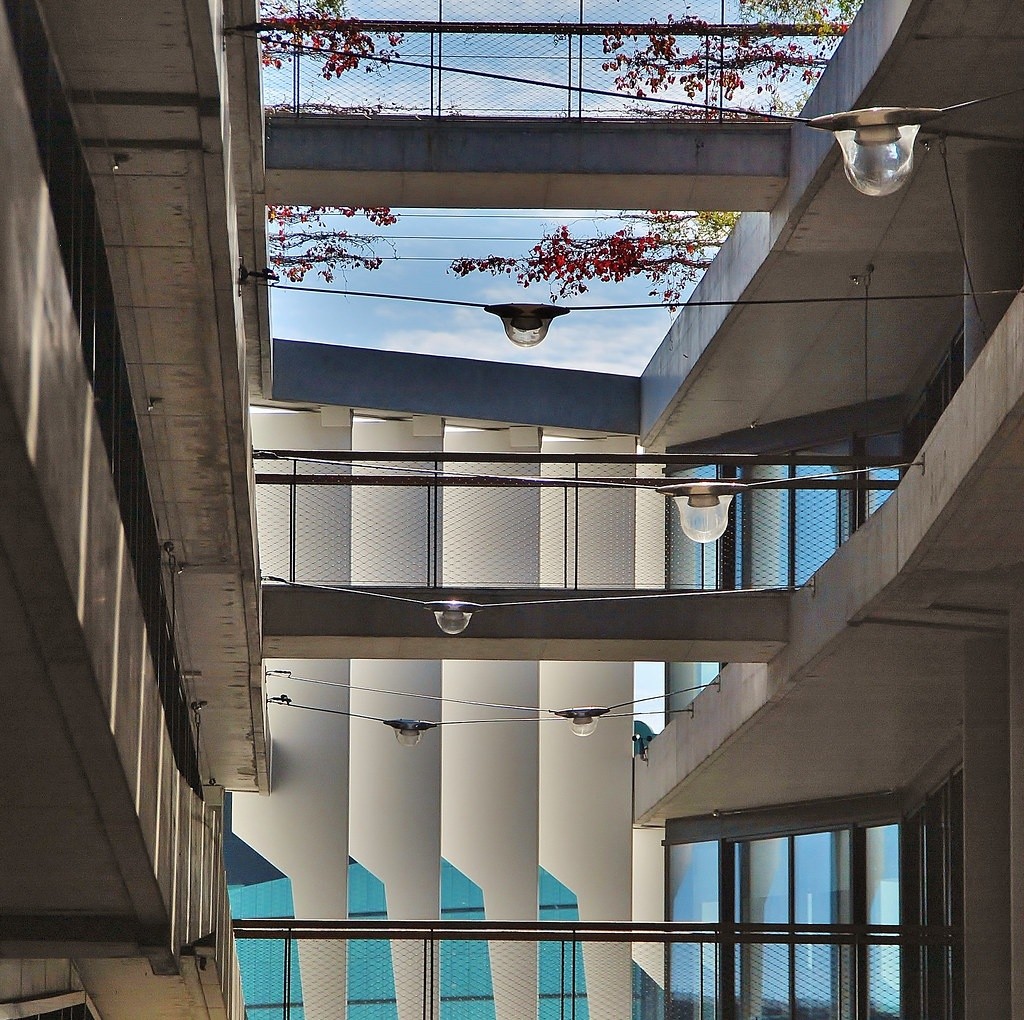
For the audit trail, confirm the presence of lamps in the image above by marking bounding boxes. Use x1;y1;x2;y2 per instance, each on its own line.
810;103;950;198
385;718;437;746
426;600;479;634
483;302;571;348
658;482;739;545
555;707;609;737
